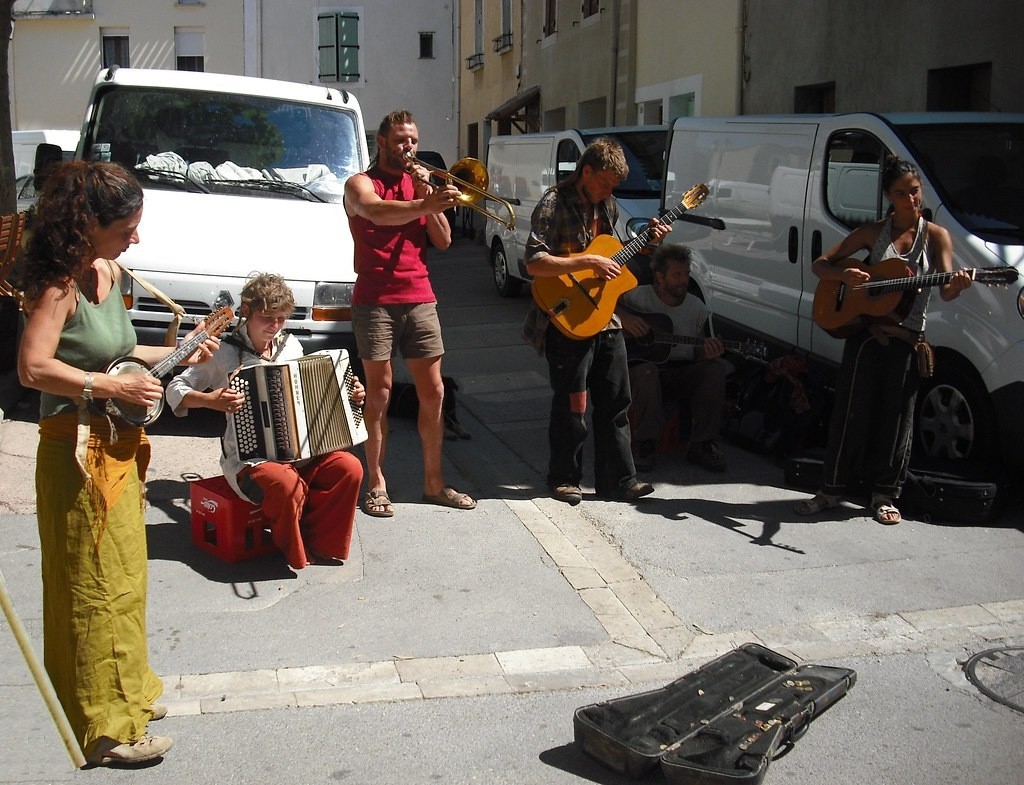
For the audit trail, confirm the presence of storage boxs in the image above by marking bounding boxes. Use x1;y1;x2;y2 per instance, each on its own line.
189;475;278;560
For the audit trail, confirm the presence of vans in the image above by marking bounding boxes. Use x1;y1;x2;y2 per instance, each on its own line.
485;126;675;303
659;112;1024;476
72;64;374;415
10;130;87;177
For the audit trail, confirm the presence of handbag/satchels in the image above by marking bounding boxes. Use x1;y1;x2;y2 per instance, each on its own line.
915;341;935;379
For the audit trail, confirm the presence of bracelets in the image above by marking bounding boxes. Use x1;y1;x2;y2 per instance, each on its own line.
83;372;92;399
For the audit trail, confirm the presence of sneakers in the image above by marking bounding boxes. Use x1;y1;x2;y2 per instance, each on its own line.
596;481;654;499
632;439;651;461
549;480;582;501
686;439;729;471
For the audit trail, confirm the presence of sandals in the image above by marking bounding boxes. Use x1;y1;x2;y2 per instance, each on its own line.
89;735;174;766
148;705;168;720
869;493;901;524
794;490;841;516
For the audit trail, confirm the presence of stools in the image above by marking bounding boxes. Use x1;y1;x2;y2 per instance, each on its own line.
628;397;683;452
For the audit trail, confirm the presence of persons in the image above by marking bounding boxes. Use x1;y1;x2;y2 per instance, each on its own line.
794;154;970;524
166;273;366;568
616;243;728;470
17;161;222;759
521;141;673;500
343;110;476;517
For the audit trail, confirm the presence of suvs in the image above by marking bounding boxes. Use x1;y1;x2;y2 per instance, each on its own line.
417;151;458;238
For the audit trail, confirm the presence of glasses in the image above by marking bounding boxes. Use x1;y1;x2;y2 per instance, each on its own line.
261;316;285;324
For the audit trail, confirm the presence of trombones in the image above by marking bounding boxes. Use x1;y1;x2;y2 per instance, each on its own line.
399;152;519;233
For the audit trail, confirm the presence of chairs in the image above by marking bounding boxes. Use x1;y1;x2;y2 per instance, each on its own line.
0;213;31;365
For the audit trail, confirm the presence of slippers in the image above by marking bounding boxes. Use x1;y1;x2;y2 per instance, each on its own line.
422;485;476;509
360;491;394;516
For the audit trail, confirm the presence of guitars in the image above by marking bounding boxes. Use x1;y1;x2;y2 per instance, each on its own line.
811;255;1021;340
529;182;711;341
611;301;769;365
95;305;236;430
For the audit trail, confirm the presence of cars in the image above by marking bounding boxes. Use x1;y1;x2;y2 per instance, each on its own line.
16;174;44;212
